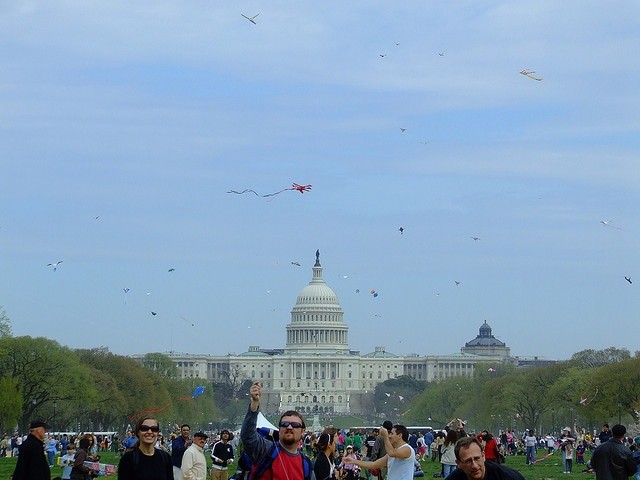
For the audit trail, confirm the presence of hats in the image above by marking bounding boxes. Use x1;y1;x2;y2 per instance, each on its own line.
30;420;52;429
194;432;209;440
347;445;352;449
383;421;394;428
563;426;572;432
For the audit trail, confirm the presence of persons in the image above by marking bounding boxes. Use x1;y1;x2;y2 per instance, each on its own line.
409;429;446;462
337;428;369;449
574;419;585;463
154;424;180;455
0;433;28;458
302;430;322;459
476;430;489;443
45;433;56;468
442;439;524;480
496;429;523;457
322;428;344;480
227;428;242;455
483;434;499;462
180;431;210;480
12;418;53;480
56;433;78;455
346;423;419;480
203;428;221;451
441;429;458;479
119;417;176;480
522;429;529;450
171;424;192;480
70;438;92;480
314;433;339;480
582;427;599;452
524;431;540;464
546;432;555;455
364;428;379;480
563;441;576;473
577;440;585;465
590;425;640;480
71;434;98;480
413;453;424;478
61;444;76;480
555;437;561;452
210;430;234;480
236;381;315;480
561;424;580;472
599;425;612;443
536;435;546;450
92;424;139;456
369;420;393;480
341;442;361;479
445;418;467;440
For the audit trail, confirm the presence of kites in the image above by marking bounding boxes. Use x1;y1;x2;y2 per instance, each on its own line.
45;260;63;273
228;181;312;202
518;67;543;84
239;10;261;28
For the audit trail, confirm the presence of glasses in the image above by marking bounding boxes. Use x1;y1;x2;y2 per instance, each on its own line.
182;430;189;431
279;421;303;428
339;451;344;453
372;430;378;433
458;452;483;465
139;425;159;432
